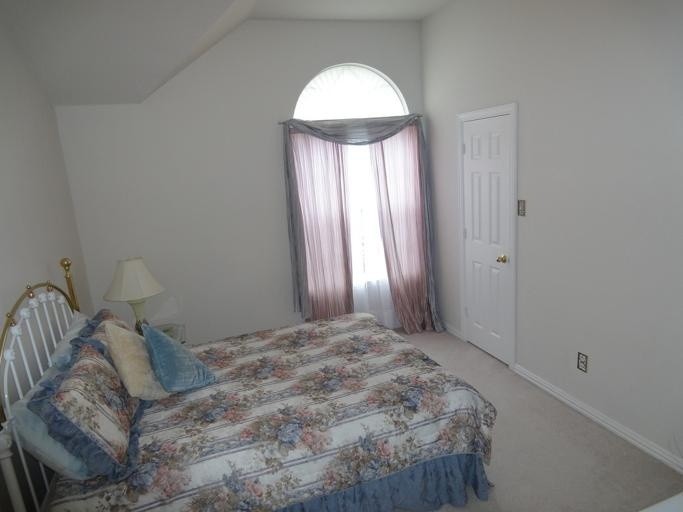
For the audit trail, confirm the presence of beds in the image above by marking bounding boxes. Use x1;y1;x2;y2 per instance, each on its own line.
0;256;498;511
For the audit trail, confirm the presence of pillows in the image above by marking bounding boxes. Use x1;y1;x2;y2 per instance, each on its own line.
48;309;94;366
141;323;215;393
14;365;99;484
102;321;174;403
27;342;140;484
72;308;134;369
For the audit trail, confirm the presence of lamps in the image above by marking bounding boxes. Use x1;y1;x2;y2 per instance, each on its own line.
101;258;167;337
